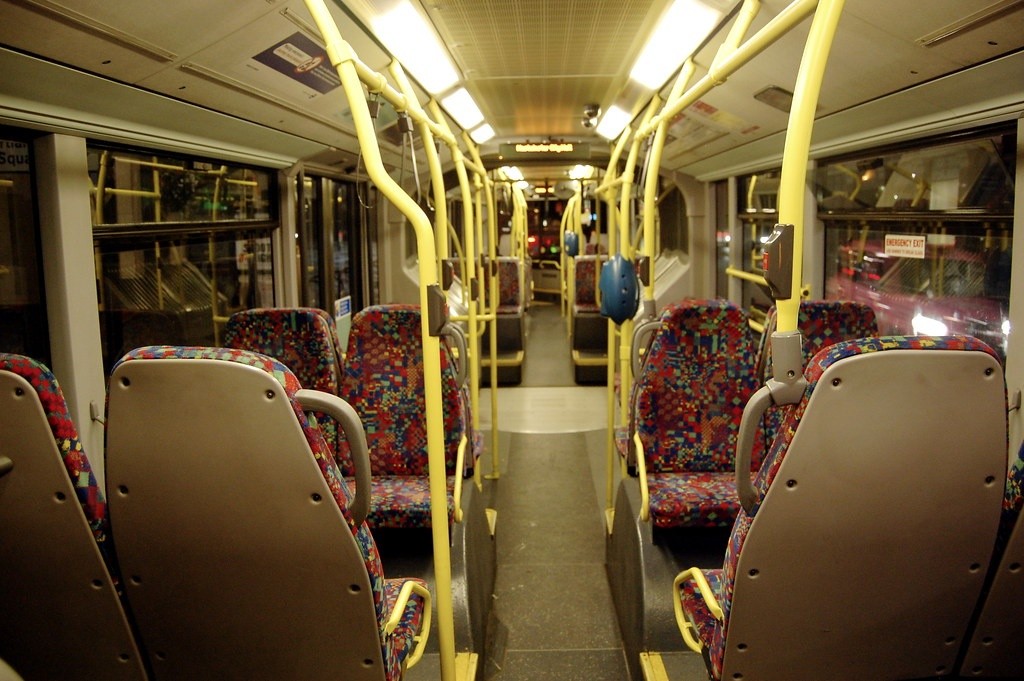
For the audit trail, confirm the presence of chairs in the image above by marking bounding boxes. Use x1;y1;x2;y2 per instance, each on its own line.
566;218;1023;681
0;251;527;681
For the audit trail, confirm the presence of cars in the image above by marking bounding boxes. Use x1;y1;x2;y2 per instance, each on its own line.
823;238;1008;362
